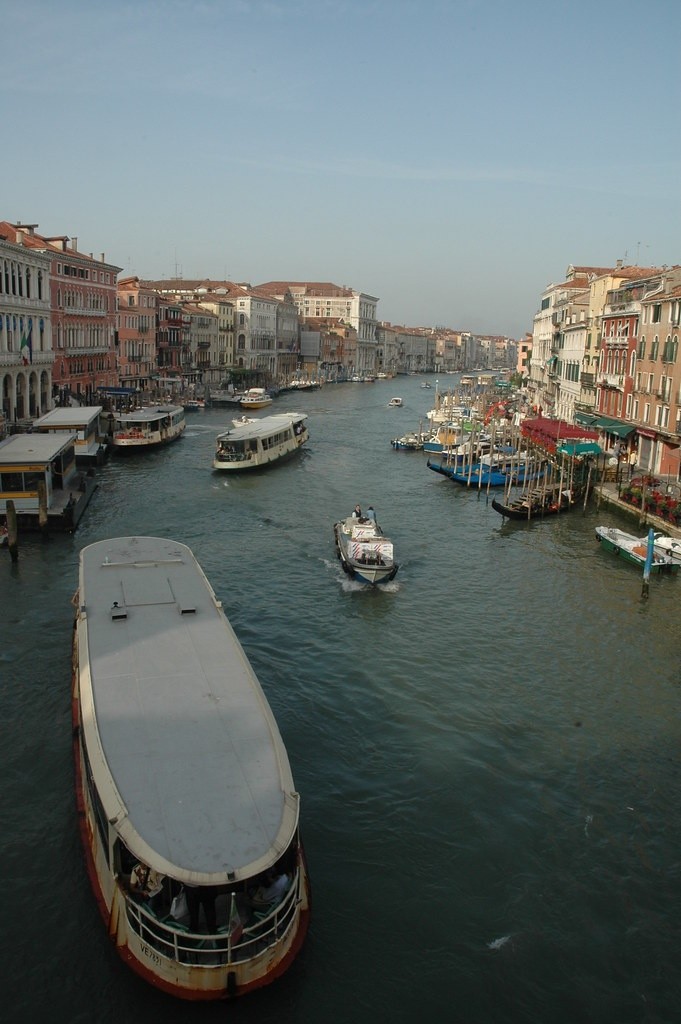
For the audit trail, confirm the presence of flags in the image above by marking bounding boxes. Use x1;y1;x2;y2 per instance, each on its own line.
20;331;32;365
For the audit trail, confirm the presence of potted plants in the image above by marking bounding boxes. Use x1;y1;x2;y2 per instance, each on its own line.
623;487;681;524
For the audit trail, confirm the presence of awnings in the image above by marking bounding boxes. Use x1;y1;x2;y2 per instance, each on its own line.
572;413;597;424
606;421;635;438
591;416;616;431
546;356;557;365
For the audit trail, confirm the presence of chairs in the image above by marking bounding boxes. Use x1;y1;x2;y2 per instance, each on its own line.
142;871;295;964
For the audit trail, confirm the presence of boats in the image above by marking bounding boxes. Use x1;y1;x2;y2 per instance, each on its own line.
595;525;681;574
639;531;681;561
210;412;310;472
240;387;273;409
391;373;618;521
387;397;402;407
112;405;186;450
69;533;312;1001
333;516;399;587
231;416;262;429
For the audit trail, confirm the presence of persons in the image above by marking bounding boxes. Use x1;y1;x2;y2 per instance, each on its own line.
352;505;361;518
217;447;253;459
561;490;574;504
628;450;638;478
297;425;302;433
242;414;246;423
366;507;376;522
129;860;292;932
613;442;621;470
